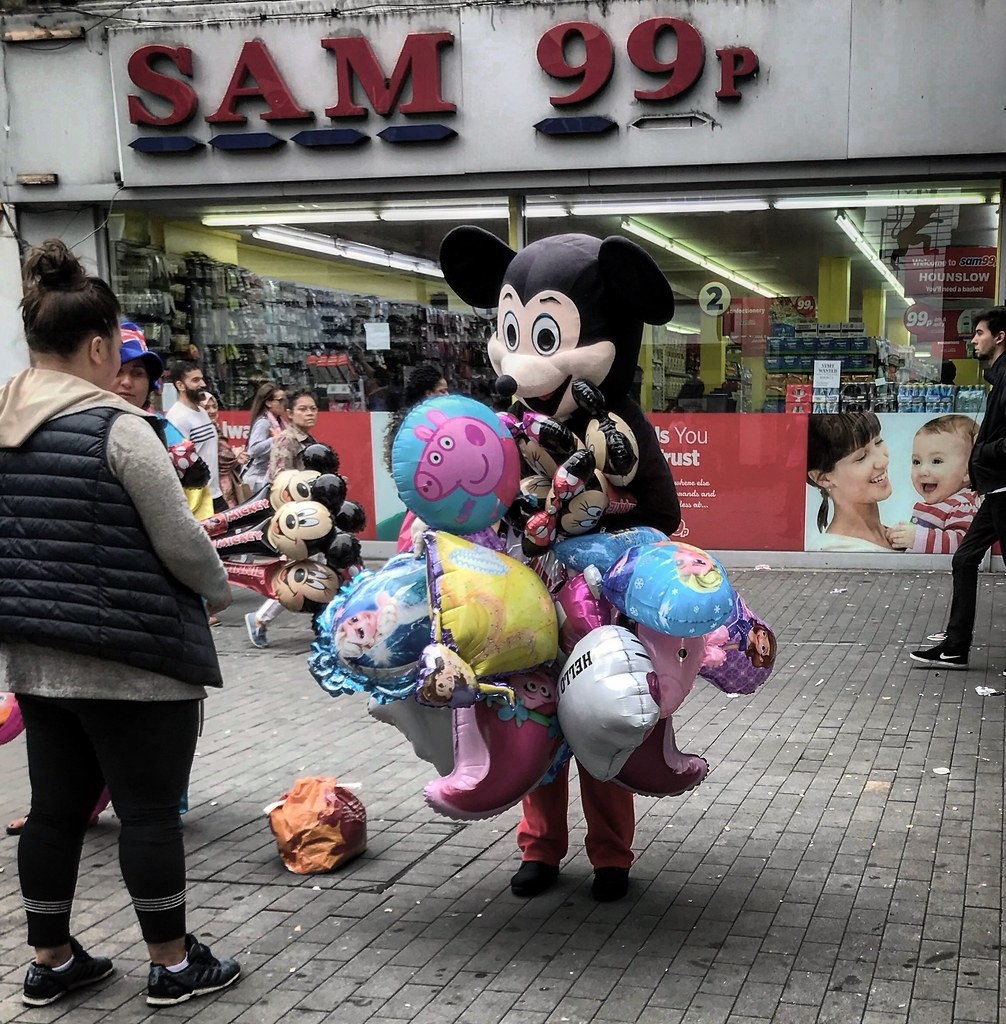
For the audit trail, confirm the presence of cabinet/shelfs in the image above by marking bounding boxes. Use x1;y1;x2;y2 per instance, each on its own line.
727;361;753;413
764;350;876;401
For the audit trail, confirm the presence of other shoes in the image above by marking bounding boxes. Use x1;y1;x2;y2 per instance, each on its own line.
243;611;269;649
7;815;32;835
592;866;629;903
512;860;560;895
209;616;221;627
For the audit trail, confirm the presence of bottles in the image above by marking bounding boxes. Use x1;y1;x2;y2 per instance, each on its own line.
898;384;994;412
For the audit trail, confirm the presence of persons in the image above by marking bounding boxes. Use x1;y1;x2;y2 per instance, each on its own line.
397;364;483;554
244;389;348;651
910;304;1006;668
0;236;289;1009
805;413;985;553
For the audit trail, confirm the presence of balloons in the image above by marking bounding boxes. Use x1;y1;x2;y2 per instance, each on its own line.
311;377;778;823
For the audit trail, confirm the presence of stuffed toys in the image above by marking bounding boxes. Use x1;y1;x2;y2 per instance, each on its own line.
438;225;675;900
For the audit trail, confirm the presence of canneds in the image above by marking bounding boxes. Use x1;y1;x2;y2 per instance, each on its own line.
786;382;956;413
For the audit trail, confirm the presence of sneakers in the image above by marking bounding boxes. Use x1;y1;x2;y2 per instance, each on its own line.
22;935;114;1006
145;932;243;1005
910;638;969;669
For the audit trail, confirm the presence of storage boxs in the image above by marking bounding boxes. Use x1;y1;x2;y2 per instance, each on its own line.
795;323;817;338
817;338;834;350
851;336;870;351
783;337;800;349
848;355;867;368
833;354;849;368
841;323;866;337
773;324;795;337
812;394;955;413
764;355;780;370
766;337;783;350
818;322;841;338
781;355;800;369
785;383;813;403
763;401;785;413
814;354;832;360
833;338;851;351
787;402;812;414
799;355;814;369
800;338;816;350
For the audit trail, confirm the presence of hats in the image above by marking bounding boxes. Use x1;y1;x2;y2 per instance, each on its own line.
119;320;163;393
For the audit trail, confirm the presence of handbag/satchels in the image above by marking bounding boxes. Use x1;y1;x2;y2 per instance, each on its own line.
263;775;369;875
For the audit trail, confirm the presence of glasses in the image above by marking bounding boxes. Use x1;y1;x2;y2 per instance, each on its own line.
291;406;320;415
273;397;287;404
